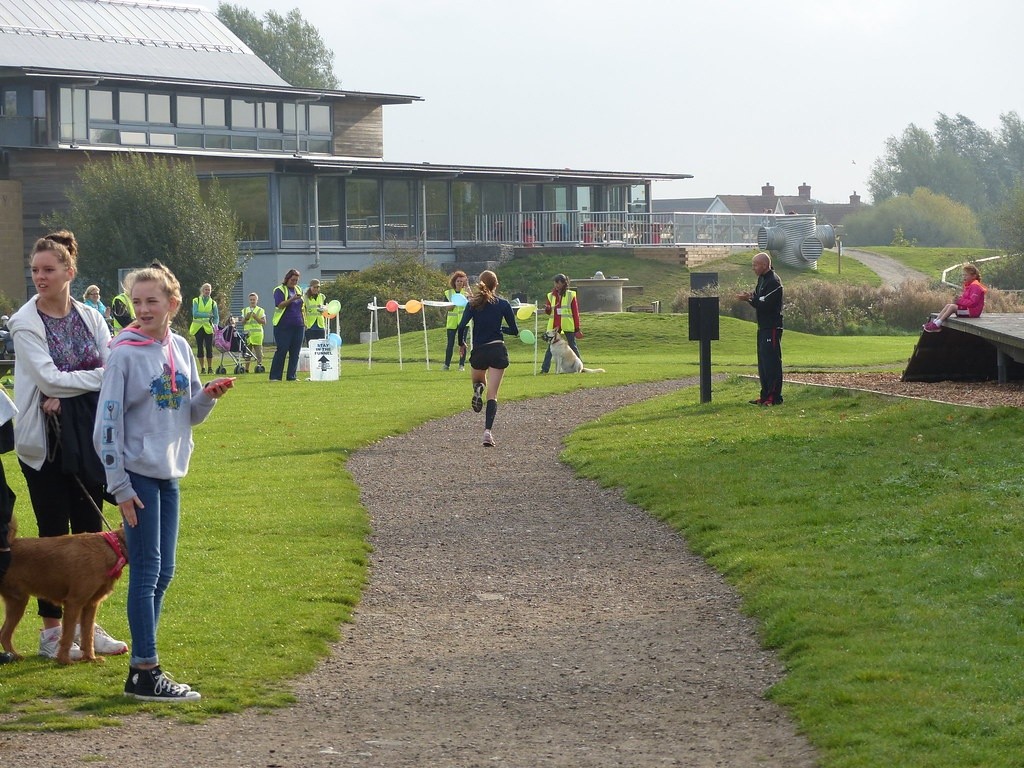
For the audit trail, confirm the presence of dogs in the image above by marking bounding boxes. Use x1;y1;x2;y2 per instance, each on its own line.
541;326;606;375
0;511;129;665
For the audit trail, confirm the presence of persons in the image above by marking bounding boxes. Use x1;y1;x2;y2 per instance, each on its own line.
269;269;305;382
6;229;128;660
458;270;519;447
0;388;19;664
302;279;327;348
442;271;473;371
540;274;583;375
92;259;235;703
112;273;136;337
83;285;110;318
921;264;988;333
190;283;219;376
0;315;10;332
735;252;784;407
241;293;266;373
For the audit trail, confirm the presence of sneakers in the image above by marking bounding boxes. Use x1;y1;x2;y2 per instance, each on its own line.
124;664;200;702
40;621;129;660
922;322;942;332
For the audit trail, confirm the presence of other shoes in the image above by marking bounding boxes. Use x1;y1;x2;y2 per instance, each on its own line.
200;368;206;375
471;383;484;413
482;432;496;446
439;365;449;371
458;365;465;372
208;368;215;375
748;398;773;407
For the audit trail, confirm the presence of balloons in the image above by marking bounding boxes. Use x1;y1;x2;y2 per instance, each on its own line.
517;306;535;320
386;300;399;313
405;300;422;314
328;333;342;346
324;300;341;319
450;293;468;307
520;330;534;344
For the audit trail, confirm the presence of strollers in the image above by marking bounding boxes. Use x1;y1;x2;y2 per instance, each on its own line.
210;315;266;375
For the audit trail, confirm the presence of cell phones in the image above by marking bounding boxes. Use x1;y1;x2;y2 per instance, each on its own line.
205;376;237;393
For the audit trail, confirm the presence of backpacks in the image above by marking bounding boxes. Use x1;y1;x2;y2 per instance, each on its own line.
213;324;234;353
